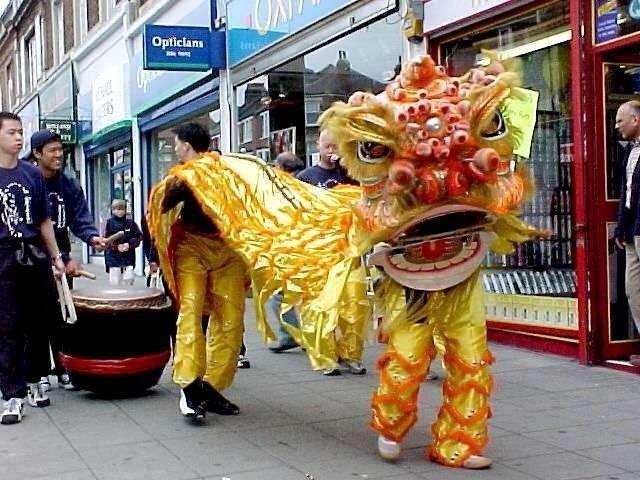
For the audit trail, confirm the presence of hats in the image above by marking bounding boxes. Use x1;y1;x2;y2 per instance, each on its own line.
31;130;57;153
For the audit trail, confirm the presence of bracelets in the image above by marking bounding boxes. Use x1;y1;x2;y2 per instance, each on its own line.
50;253;63;262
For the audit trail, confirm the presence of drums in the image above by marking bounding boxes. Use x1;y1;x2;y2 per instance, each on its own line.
57;284;177;397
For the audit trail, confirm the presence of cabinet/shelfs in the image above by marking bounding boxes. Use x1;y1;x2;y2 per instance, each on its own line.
479;99;576;294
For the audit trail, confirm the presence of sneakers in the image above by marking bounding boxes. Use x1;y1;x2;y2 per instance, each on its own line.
237;352;249;368
201;392;238;415
1;371;74;424
179;389;206;419
464;454;493;469
340;357;366;375
378;431;402;459
269;340;293;350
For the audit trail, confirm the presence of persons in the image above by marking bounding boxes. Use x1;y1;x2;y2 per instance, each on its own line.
296;128;367;376
371;275;491;468
158;121;238;421
208;148;223;155
263;151;304;350
141;209;254;372
31;129;108;391
104;200;143;284
611;100;639;362
0;112;64;424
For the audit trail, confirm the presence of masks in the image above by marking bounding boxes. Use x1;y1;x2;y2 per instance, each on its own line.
112;208;126;218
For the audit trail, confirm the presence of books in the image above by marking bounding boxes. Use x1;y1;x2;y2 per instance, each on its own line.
477;110;625;299
